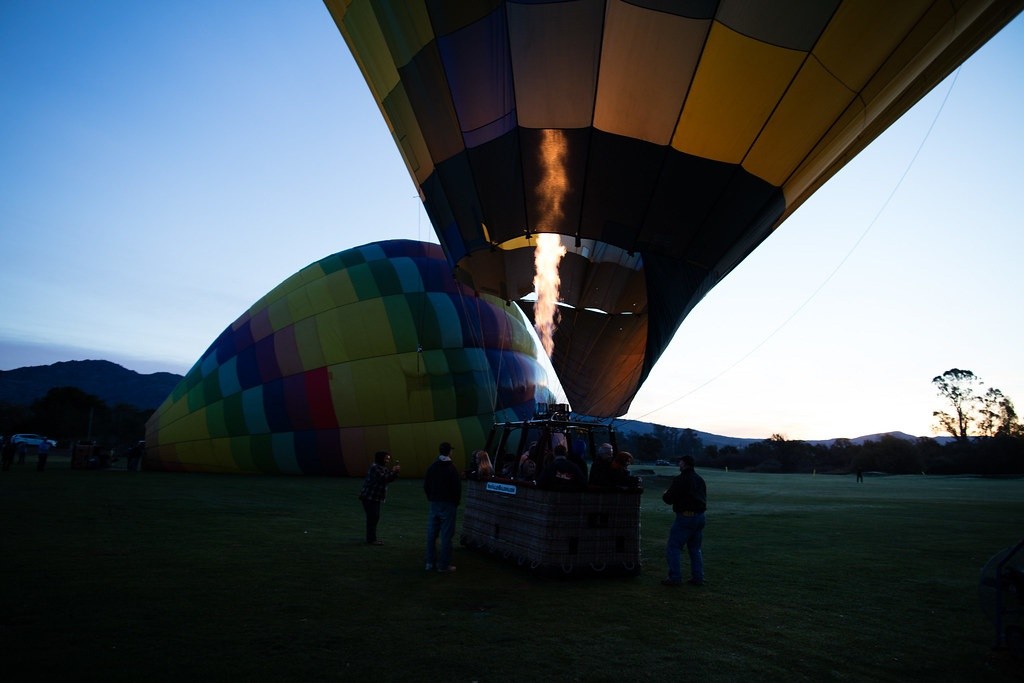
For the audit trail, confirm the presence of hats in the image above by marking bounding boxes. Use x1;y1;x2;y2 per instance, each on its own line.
439;442;455;450
684;454;696;466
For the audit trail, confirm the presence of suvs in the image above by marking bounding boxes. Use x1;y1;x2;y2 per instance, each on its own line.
10;434;57;447
655;460;670;466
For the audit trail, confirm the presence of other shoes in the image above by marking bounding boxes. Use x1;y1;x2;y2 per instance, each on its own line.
424;562;433;570
368;540;385;545
687;576;704;585
437;564;456;572
661;575;684;586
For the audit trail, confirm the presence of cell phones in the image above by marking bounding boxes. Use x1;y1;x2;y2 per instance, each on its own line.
395;460;399;465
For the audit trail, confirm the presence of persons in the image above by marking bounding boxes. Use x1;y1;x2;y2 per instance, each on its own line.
424;442;462;572
18;444;27;464
36;436;49;471
660;455;707;586
126;444;141;472
471;440;643;487
357;451;401;545
856;467;864;483
0;440;16;470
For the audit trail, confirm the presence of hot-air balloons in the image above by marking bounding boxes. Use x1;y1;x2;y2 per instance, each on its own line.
72;240;537;479
323;0;1024;579
